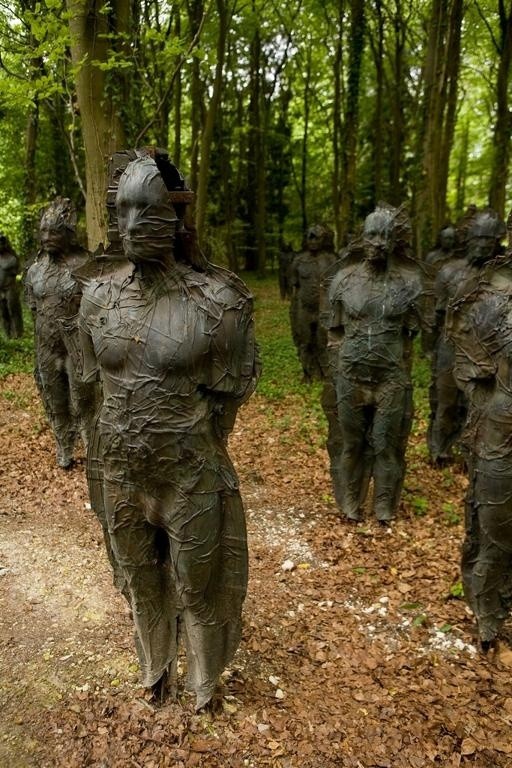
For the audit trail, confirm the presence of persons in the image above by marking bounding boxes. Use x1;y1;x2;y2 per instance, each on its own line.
276;224;341;382
72;149;258;714
0;235;28;344
21;200;94;474
419;205;512;657
317;203;432;528
